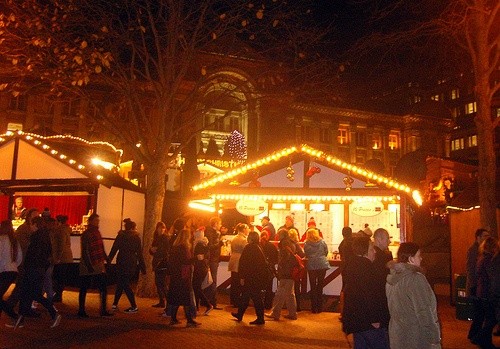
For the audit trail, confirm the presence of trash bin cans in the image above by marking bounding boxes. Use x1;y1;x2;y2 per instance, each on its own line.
454;272;473;321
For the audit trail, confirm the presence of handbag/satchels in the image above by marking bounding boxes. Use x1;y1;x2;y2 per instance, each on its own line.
266;263;277;280
456;302;475;321
1;280;20;304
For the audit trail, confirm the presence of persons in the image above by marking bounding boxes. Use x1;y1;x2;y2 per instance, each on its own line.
0;207;74;329
385;242;442;349
339;223;394;349
148;214;228;327
228;215;331;325
467;229;500;349
78;213;114;318
108;218;146;312
11;195;27;219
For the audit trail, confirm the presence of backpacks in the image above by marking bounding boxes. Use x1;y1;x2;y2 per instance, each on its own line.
282;246;305;280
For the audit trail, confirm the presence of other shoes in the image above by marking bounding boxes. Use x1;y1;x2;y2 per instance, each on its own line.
170;320;182;324
53;296;62;302
47;305;59;312
283;314;297;320
77;312;89;317
50;314;62;328
5;322;24;328
154;302;166;308
100;312;113;317
249;319;265;325
231;311;242;321
161;312;172;318
213;305;223;310
31;305;40;311
185;320;201;327
113;305;119;311
264;313;280;320
18;308;41;317
124;307;140;314
204;305;213;314
